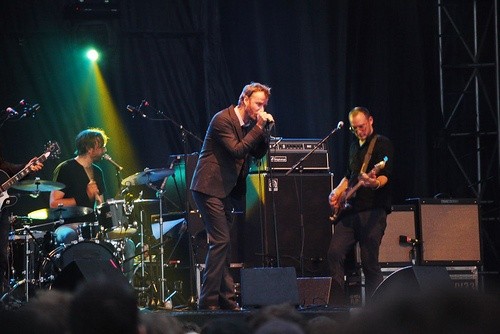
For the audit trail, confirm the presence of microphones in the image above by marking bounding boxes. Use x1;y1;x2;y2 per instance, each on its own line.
142;100;162;115
332;121;344;133
121;187;129;196
400;235;418;243
127;105;147;118
102;153;124;170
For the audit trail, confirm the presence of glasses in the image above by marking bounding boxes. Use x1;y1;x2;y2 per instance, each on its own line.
349;122;370;132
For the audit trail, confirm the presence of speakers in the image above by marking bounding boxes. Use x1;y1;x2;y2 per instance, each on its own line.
53;258;138;312
264;172;335;276
239;266;297;308
352;204;418;267
297;275;349;311
419;199;483;265
372;268;457;326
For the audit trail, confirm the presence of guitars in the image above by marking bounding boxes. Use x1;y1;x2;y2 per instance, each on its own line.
0;140;62;213
327;156;388;225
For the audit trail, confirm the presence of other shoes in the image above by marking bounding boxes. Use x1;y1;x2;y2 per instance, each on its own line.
225;305;245;312
200;306;217;311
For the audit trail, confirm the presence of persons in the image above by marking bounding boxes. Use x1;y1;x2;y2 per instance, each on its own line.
247;282;500;334
0;157;44;172
327;107;394;307
49;129;135;279
190;83;274;312
0;276;186;334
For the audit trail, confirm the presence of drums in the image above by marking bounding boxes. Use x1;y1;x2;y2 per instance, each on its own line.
97;199;140;239
11;215;56;233
37;241;124;291
7;230;58;289
77;221;127;253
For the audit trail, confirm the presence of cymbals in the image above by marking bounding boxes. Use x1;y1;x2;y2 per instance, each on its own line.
121;197;168;207
28;206;93;219
10;179;66;192
120;166;175;186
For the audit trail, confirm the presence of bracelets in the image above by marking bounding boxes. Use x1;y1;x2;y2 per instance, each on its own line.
373;181;380;190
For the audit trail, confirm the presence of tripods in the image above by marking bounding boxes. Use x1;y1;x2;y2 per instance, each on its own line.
0;172;196;314
